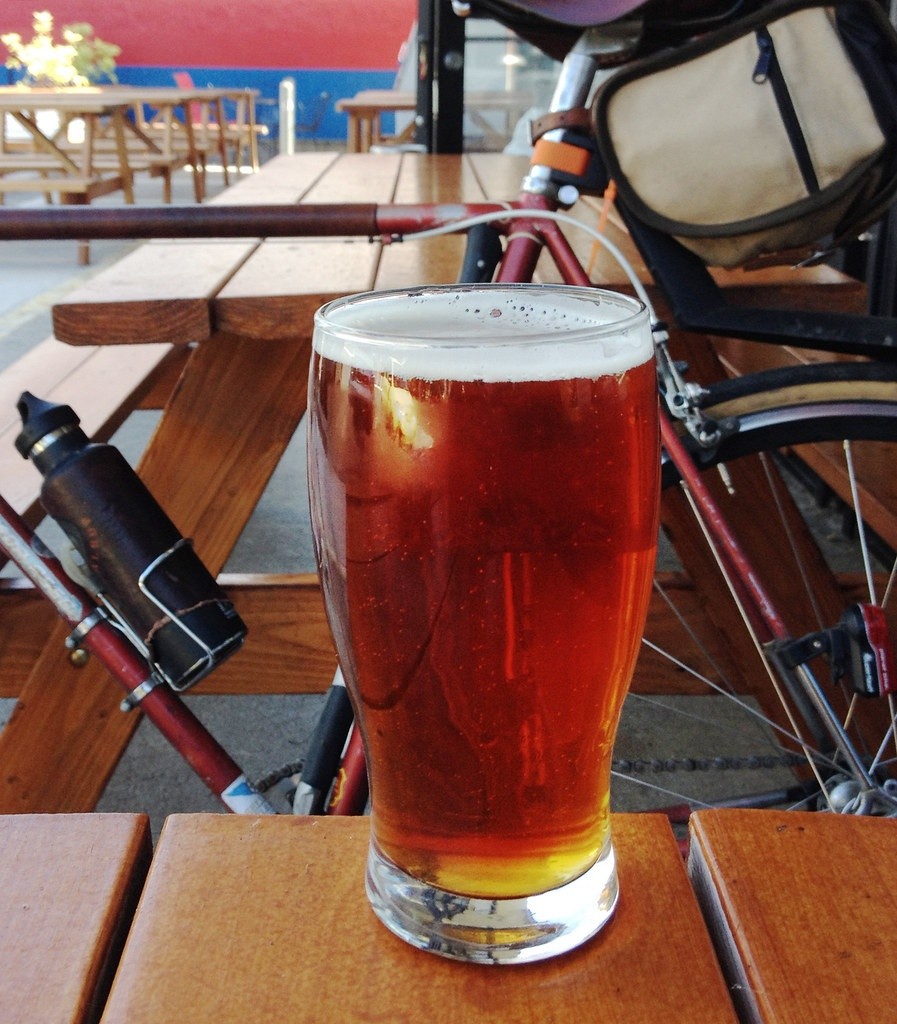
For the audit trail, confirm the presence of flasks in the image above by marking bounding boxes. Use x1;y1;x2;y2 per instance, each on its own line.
12;387;248;698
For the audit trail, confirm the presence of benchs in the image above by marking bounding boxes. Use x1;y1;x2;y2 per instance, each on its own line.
202;91;233;204
0;121;269;582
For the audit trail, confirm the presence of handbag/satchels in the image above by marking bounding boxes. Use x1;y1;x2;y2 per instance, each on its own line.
593;0;897;270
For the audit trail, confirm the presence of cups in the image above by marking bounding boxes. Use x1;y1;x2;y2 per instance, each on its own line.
307;281;661;967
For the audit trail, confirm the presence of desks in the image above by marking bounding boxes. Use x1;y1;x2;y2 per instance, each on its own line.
354;87;523;147
335;97;530;150
0;100;136;267
0;151;897;818
136;95;201;206
230;87;263;174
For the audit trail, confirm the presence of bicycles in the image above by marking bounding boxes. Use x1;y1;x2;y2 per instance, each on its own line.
0;0;897;851
